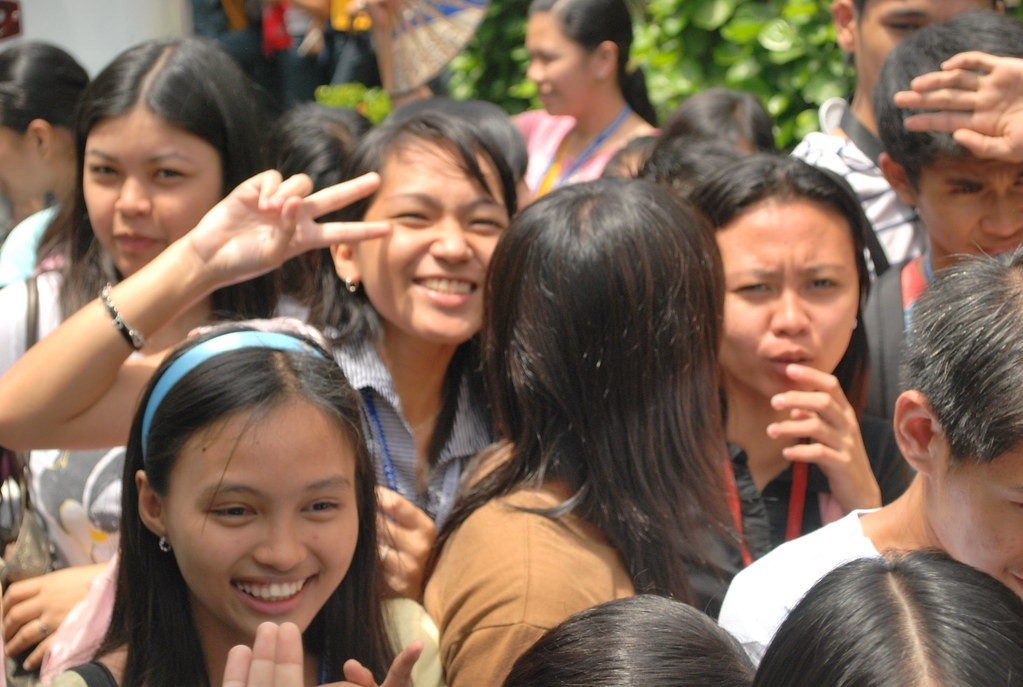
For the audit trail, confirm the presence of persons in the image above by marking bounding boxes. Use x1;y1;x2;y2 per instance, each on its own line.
0;0;1023;687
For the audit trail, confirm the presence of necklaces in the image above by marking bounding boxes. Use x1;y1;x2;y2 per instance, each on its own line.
364;394;401;492
534;104;630;194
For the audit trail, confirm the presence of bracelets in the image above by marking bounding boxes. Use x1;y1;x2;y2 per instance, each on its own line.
99;289;146;352
